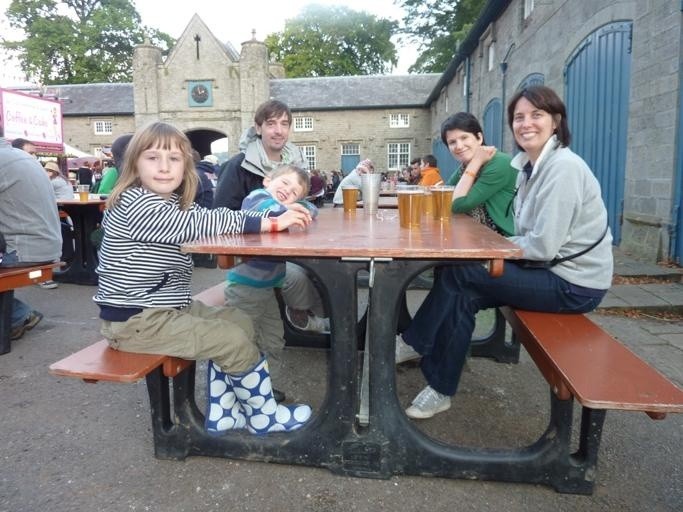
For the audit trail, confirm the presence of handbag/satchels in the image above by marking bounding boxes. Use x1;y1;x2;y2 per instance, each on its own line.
515;254;555;270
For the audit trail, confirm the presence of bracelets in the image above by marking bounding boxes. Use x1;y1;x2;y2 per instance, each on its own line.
464;170;477;178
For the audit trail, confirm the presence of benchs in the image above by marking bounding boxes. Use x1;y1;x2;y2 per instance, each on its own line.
0;260;69;355
45;275;292;464
462;299;683;497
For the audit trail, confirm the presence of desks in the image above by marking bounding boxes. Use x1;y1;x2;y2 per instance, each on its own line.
176;175;526;485
53;190;116;287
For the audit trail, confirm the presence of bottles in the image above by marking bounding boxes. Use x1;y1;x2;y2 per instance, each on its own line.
385;176;397;191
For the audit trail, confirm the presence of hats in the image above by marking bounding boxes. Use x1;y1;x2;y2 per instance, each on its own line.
355;157;374;174
43;162;65;176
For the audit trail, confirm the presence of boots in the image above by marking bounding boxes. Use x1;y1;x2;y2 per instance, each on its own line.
227;352;313;436
204;358;247;433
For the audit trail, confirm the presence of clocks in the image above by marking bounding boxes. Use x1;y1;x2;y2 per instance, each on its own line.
190;82;209;105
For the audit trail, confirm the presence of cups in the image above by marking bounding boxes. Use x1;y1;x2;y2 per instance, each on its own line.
395;184;454;231
339;184;358;213
359;174;384;215
76;185;90;201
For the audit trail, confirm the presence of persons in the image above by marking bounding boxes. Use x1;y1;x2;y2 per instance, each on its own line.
395;85;614;419
441;112;519;238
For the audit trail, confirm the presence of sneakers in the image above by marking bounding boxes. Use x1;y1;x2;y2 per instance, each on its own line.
284;303;333;335
36;277;58;290
393;332;430;363
8;308;43;341
402;383;452;420
